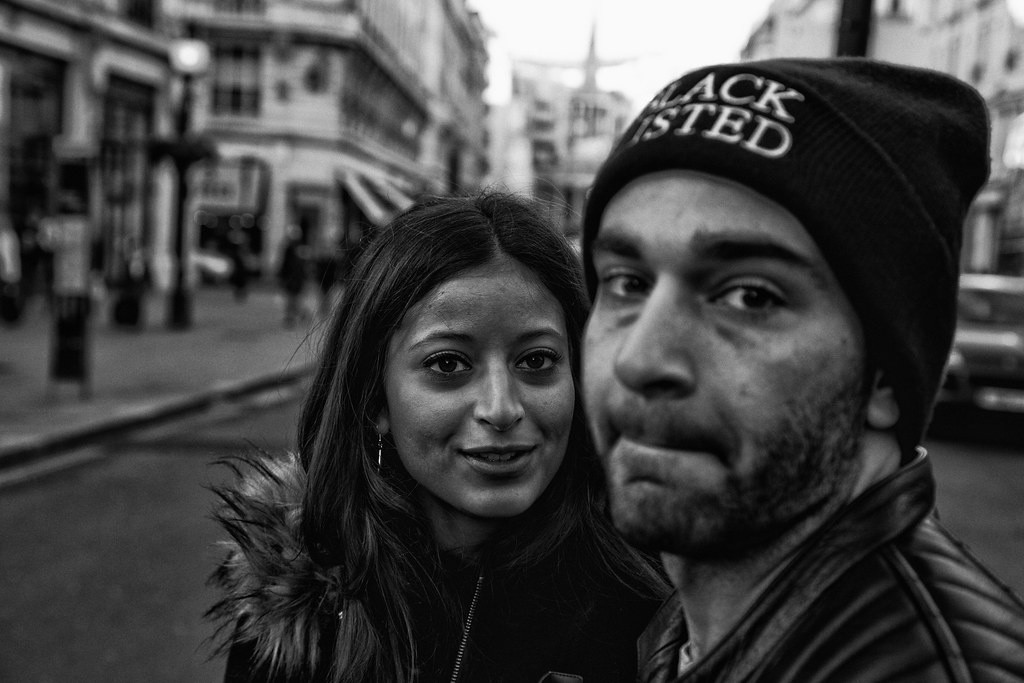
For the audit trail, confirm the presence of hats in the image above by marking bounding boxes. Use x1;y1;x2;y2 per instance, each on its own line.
581;57;993;460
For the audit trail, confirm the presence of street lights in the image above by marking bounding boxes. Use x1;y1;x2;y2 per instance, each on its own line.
168;40;210;330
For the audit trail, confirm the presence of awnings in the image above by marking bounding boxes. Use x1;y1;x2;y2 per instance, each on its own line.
341;168;417;227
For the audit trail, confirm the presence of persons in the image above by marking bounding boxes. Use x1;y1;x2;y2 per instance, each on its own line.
0;190;679;683
571;55;1024;683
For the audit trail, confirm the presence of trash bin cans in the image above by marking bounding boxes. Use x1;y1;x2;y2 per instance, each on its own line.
44;269;105;378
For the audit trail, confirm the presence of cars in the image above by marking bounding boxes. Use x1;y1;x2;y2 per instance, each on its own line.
865;273;1024;435
